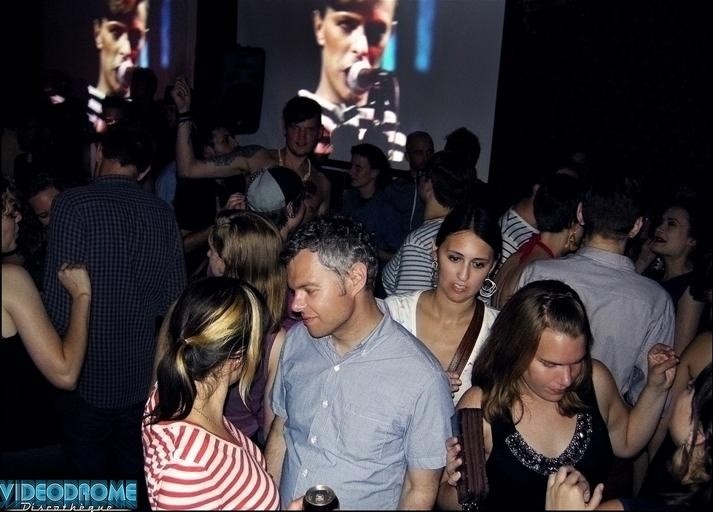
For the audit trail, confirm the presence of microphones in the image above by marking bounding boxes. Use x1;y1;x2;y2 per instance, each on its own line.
117;60;137;87
346;61;395;93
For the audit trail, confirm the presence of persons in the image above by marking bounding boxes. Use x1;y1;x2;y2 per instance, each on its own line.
2;74;713;511
294;1;410;165
53;1;156;134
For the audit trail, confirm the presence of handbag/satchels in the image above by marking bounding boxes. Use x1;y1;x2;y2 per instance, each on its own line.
450;408;489;510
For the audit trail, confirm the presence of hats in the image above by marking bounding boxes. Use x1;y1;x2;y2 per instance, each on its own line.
247;167;317;215
